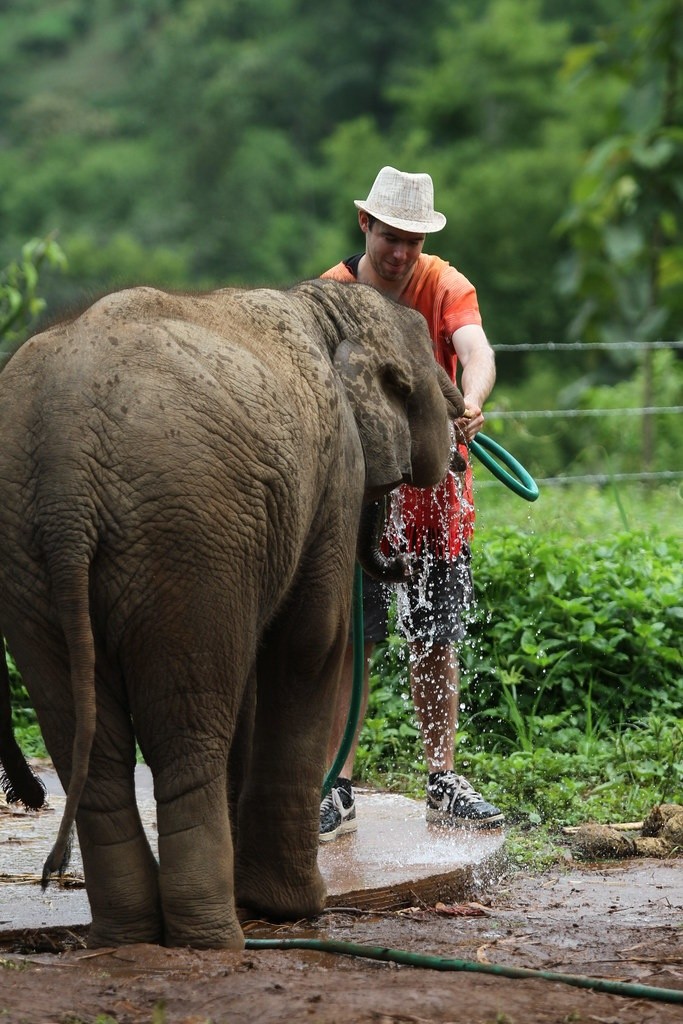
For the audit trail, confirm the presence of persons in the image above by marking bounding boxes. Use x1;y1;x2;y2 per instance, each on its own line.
306;163;506;843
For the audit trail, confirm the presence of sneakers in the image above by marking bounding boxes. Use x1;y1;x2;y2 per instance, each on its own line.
318;787;358;841
425;774;505;828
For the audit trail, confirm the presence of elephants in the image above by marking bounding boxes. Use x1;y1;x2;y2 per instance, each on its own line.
1;280;463;938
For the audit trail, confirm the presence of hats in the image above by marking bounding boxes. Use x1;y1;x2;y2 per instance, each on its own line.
353;166;446;233
